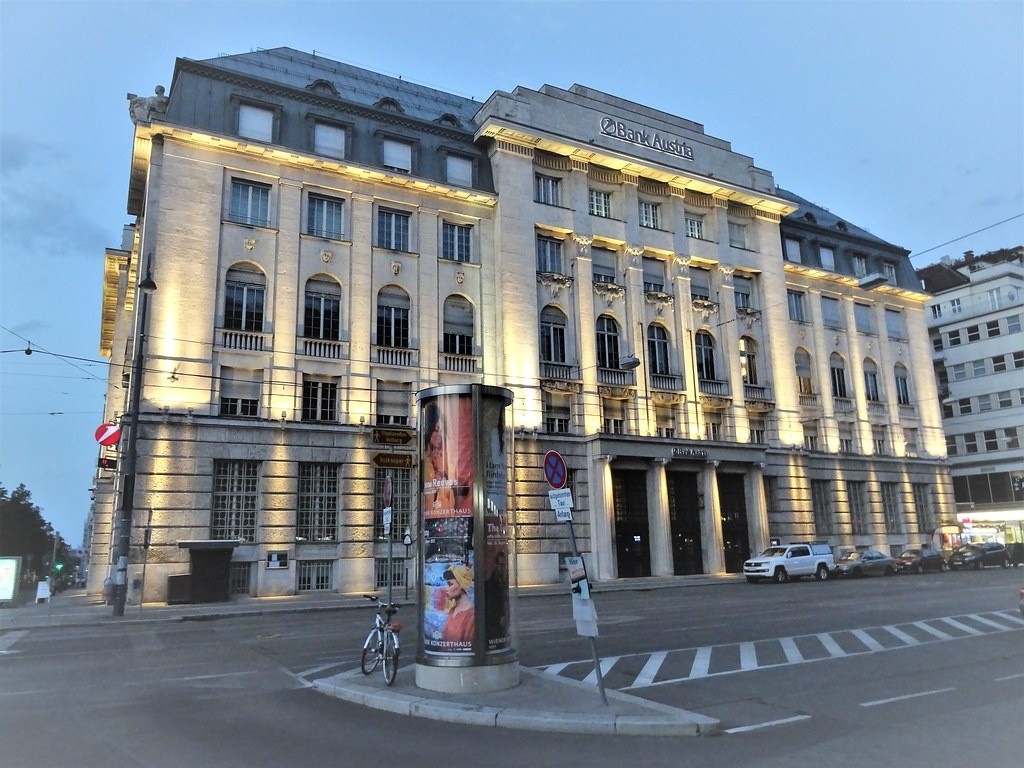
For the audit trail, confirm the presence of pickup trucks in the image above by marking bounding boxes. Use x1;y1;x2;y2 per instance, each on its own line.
743;544;837;584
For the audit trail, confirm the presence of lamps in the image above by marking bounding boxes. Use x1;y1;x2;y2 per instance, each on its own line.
620;357;640;370
521;425;524;431
360;415;365;425
188;407;193;414
858;271;888;291
164;405;170;412
281;411;286;420
534;425;538;432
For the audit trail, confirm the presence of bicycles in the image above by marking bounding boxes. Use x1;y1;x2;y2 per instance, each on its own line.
361;595;403;687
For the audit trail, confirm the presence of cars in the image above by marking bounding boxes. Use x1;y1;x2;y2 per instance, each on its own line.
835;549;897;579
946;541;1012;570
894;548;948;575
